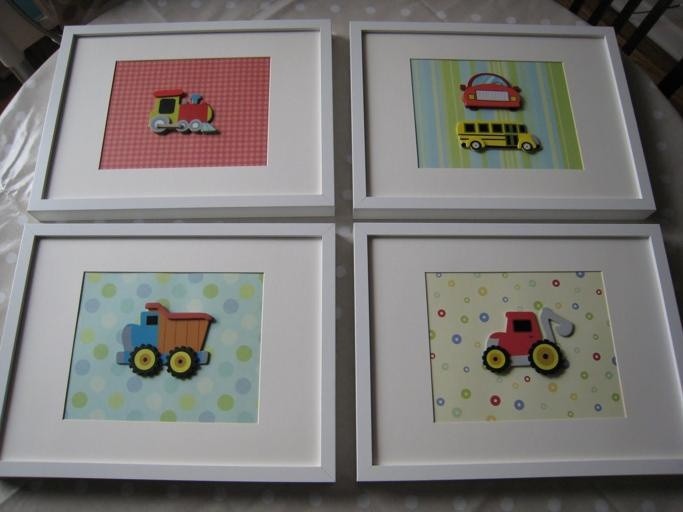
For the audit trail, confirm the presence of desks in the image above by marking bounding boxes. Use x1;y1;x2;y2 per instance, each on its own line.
0;2;683;508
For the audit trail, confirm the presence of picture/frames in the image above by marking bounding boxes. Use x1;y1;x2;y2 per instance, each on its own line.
26;17;332;221
1;222;338;478
350;23;657;220
350;219;683;479
